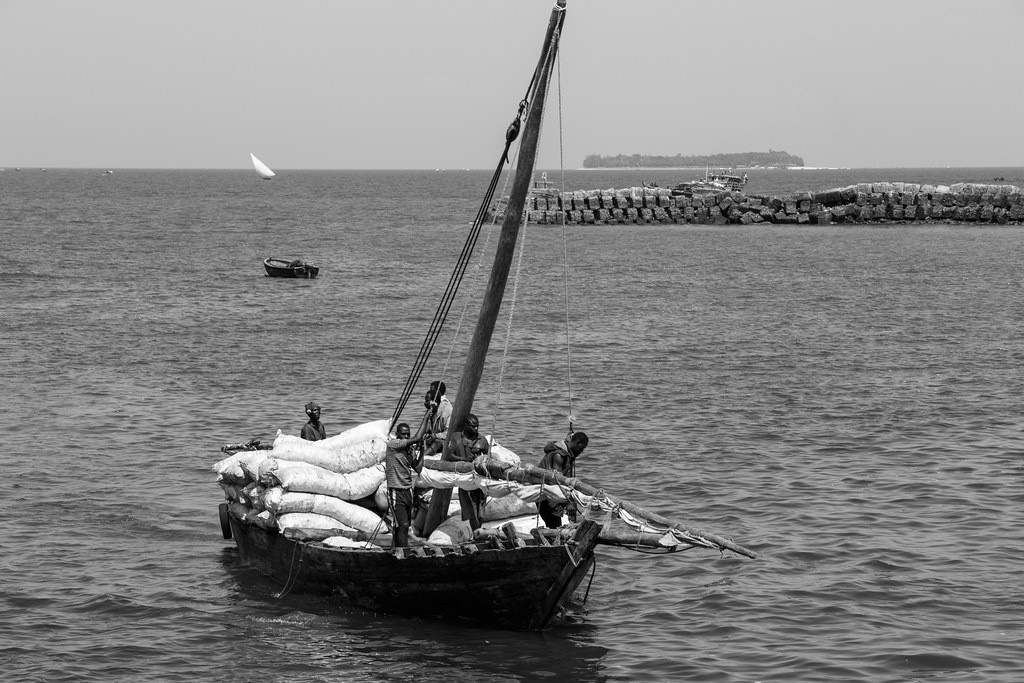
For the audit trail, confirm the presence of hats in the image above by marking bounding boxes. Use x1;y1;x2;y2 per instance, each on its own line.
305;402;320;415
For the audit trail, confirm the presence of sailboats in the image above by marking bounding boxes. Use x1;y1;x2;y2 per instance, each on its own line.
214;1;601;629
250;153;277;181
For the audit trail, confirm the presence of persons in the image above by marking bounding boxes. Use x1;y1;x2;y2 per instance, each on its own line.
386;409;433;548
424;381;453;456
447;414;490;532
533;432;589;529
301;402;326;441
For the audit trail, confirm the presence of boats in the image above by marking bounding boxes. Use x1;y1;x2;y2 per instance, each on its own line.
641;165;747;199
261;255;318;279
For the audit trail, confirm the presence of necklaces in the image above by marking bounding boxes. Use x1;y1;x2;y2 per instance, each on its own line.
460;432;478;456
